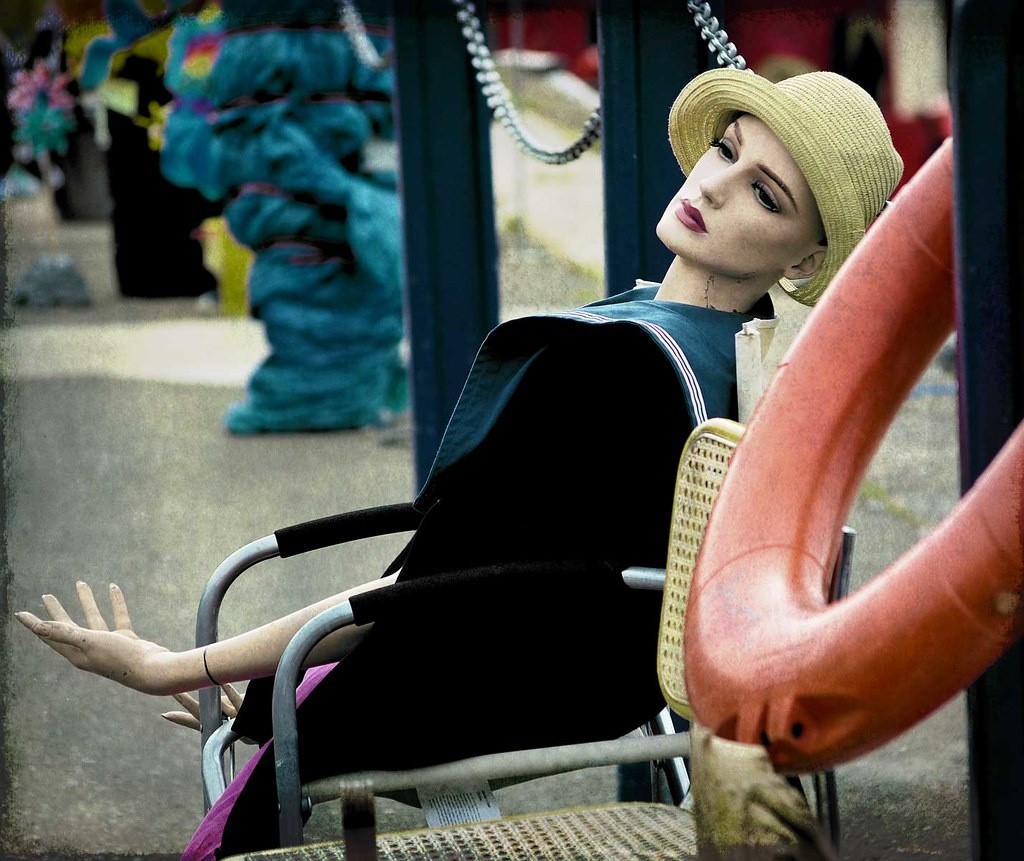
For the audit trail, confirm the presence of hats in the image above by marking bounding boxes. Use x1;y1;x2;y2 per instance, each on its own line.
668;68;905;307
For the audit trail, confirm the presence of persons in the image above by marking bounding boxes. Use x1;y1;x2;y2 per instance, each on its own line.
13;65;905;861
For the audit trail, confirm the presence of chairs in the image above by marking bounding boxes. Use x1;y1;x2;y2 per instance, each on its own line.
195;407;857;860
